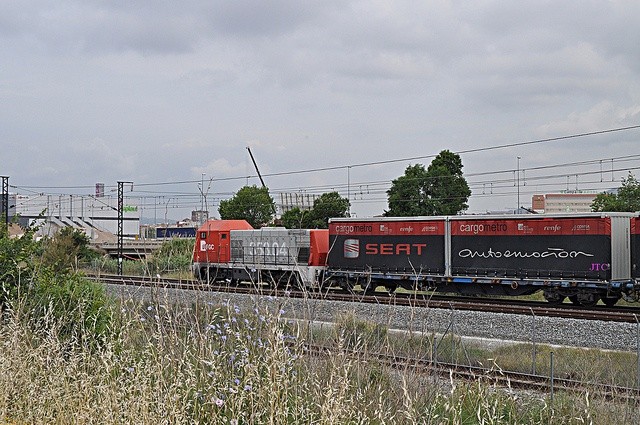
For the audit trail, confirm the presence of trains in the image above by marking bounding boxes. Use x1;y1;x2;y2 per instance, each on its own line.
189;211;640;307
